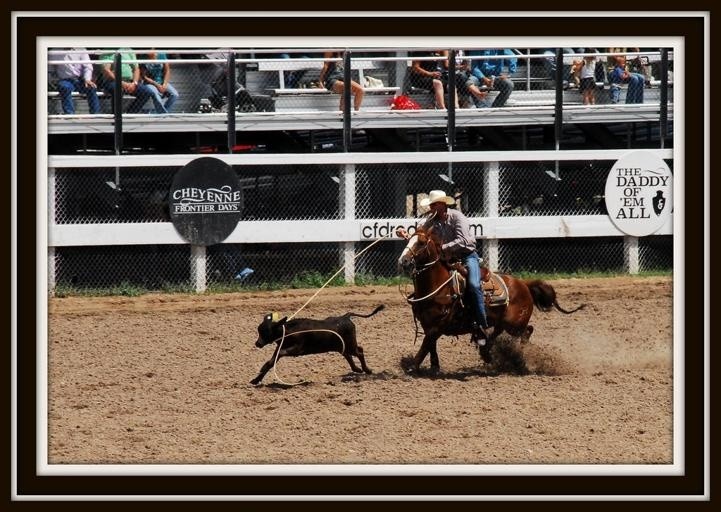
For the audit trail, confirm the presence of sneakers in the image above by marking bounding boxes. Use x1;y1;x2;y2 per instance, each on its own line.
473;321;486;346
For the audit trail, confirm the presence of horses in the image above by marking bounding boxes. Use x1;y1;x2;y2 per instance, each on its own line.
396;223;586;375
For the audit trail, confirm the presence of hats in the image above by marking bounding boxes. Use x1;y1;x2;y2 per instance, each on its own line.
420;190;455;207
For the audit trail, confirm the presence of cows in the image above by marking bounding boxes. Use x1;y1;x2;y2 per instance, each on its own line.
250;303;385;386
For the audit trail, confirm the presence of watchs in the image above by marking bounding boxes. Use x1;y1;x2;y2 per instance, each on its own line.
133;81;138;86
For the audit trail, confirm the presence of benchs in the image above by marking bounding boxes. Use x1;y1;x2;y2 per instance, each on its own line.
561;52;674;87
408;60;492;91
49;89;137;100
256;61;402;94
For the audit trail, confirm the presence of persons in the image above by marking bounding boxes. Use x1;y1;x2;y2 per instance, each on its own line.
538;47;674;104
318;52;365;111
410;51;461;109
397;189;488;347
438;50;495;109
468;50;520;107
141;47;179;114
48;48;101;115
99;48;152;114
278;53;310;88
